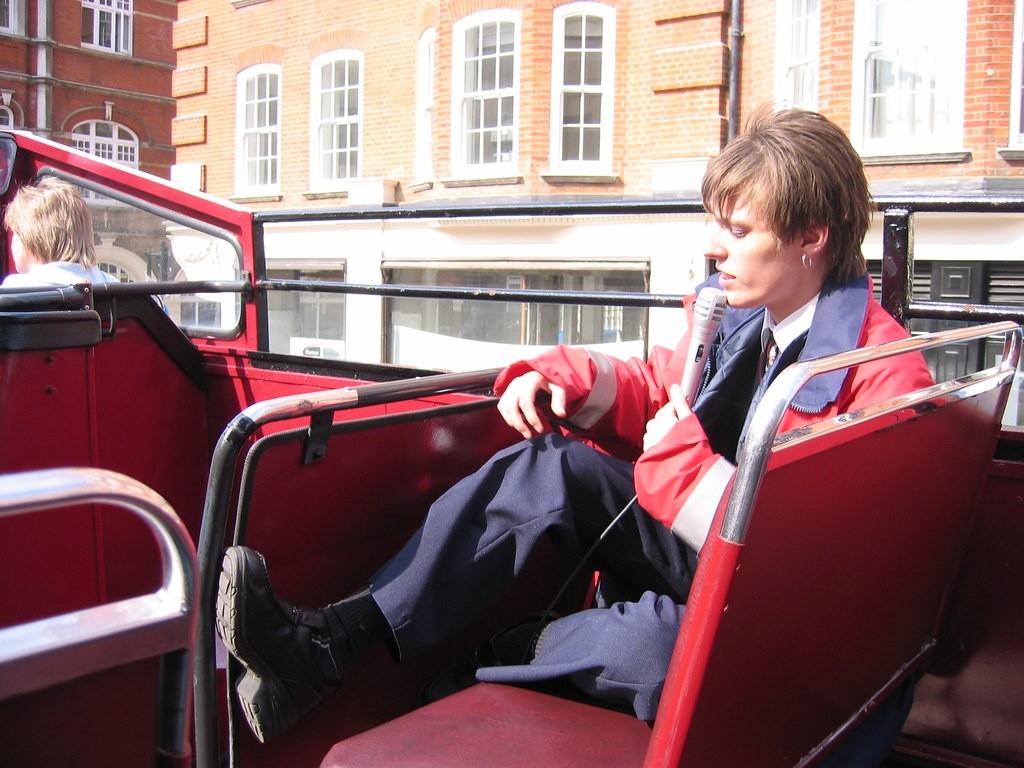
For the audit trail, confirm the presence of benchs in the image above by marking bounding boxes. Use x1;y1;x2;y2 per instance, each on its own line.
0;315;1023;767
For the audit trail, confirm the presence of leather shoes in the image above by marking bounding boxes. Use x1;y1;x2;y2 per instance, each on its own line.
216;545;355;744
419;608;561;705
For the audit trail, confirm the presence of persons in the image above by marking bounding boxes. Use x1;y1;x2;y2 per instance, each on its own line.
213;105;938;744
0;175;122;289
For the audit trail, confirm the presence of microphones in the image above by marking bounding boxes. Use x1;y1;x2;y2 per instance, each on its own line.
679;287;729;409
